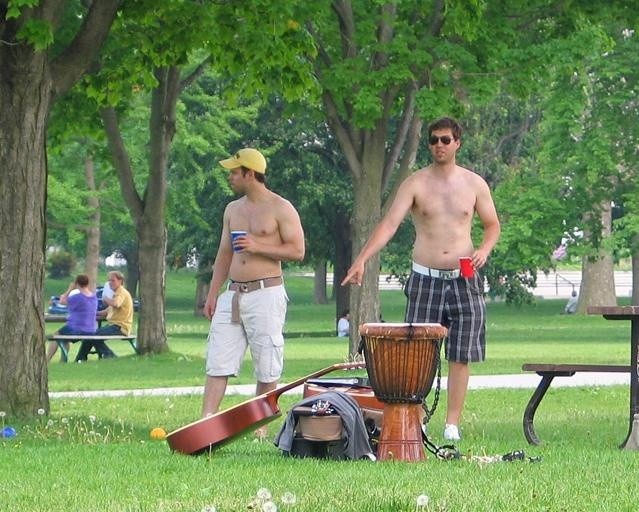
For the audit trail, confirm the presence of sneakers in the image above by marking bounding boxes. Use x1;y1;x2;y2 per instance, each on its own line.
445;425;459;439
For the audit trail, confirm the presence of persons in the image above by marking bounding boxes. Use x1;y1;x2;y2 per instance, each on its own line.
561;291;578;314
341;117;500;442
199;147;305;419
45;273;97;363
72;271;133;362
337;309;350;339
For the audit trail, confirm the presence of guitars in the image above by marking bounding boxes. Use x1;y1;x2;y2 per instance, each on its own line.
302;375;385;416
165;360;368;455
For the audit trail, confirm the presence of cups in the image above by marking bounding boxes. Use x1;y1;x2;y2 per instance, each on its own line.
458;256;473;278
231;231;246;251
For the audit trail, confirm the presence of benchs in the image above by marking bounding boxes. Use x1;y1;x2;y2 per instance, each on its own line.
522;364;631;445
46;334;141;361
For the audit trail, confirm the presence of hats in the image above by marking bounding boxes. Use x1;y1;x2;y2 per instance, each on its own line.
219;149;266;174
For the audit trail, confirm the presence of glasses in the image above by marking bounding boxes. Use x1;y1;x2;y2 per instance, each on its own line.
427;136;451;144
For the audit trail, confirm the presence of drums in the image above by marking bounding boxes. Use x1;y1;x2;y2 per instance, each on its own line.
358;323;448;462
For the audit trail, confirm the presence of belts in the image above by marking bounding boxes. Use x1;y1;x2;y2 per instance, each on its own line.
411;261;460;280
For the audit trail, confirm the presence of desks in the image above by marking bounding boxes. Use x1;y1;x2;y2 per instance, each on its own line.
43;311;108;329
587;305;639;447
